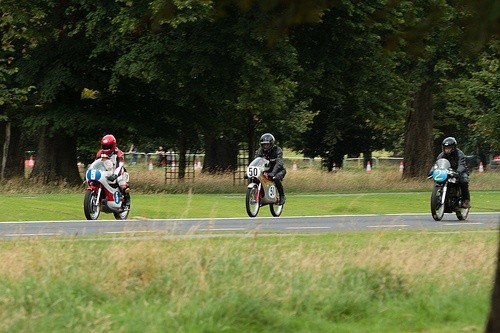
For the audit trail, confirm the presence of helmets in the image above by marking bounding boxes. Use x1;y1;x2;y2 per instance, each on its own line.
101;134;116;154
259;133;275;151
441;137;457;155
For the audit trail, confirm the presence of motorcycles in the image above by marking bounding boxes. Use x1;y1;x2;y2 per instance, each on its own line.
246;156;285;218
424;159;471;221
84;158;131;220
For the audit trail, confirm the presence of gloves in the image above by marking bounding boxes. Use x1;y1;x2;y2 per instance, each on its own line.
428;173;433;179
453;172;459;177
268;173;274;178
108;175;117;181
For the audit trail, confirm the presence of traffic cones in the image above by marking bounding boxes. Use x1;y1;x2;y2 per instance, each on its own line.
477;162;484;174
366;161;371;174
28;155;33;167
192;159;202;172
171;160;176;174
148;160;153;173
399;162;403;172
292;161;298;171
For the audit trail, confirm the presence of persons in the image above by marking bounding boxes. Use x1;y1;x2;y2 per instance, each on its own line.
129;143;138;165
428;137;471;208
244;133;287;206
92;134;131;206
156;145;175;167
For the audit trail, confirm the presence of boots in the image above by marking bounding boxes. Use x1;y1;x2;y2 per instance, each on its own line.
122;187;130;206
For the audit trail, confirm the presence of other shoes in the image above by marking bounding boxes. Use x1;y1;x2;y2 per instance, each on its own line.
279;196;285;204
462;200;470;207
